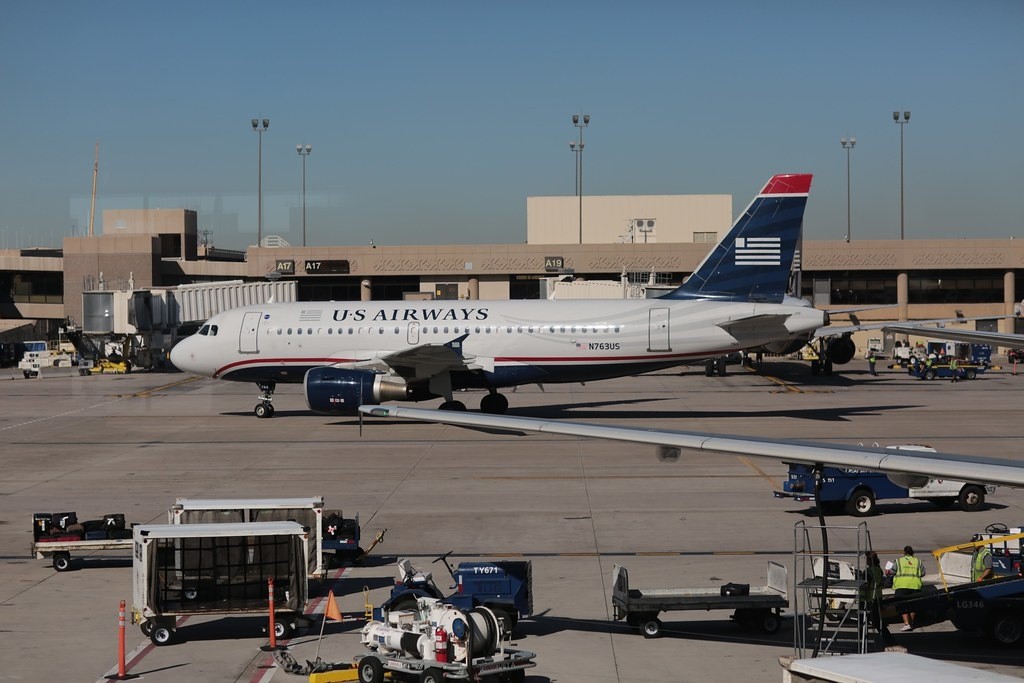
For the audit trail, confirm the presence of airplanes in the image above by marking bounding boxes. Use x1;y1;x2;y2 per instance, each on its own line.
166;173;1016;419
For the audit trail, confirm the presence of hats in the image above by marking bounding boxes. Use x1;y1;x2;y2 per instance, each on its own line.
970;534;983;542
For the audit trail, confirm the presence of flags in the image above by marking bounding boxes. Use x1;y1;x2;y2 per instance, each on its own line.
326;592;343;623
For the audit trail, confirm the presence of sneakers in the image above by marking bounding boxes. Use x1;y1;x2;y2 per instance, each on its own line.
911;621;918;628
899;625;911;631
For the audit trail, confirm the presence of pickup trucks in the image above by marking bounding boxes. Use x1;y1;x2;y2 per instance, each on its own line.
772;442;995;517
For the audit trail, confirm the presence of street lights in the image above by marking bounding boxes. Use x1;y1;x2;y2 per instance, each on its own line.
296;143;311;248
572;114;590;244
251;118;270;250
570;141;585;195
893;111;912;239
840;137;857;242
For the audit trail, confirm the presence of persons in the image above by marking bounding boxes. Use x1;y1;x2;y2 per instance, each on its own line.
949;357;960;383
864;350;876;377
889;545;926;631
920;354;933;373
62;349;66;354
756;353;762;364
863;551;884;626
970;534;993;583
902;339;905;347
